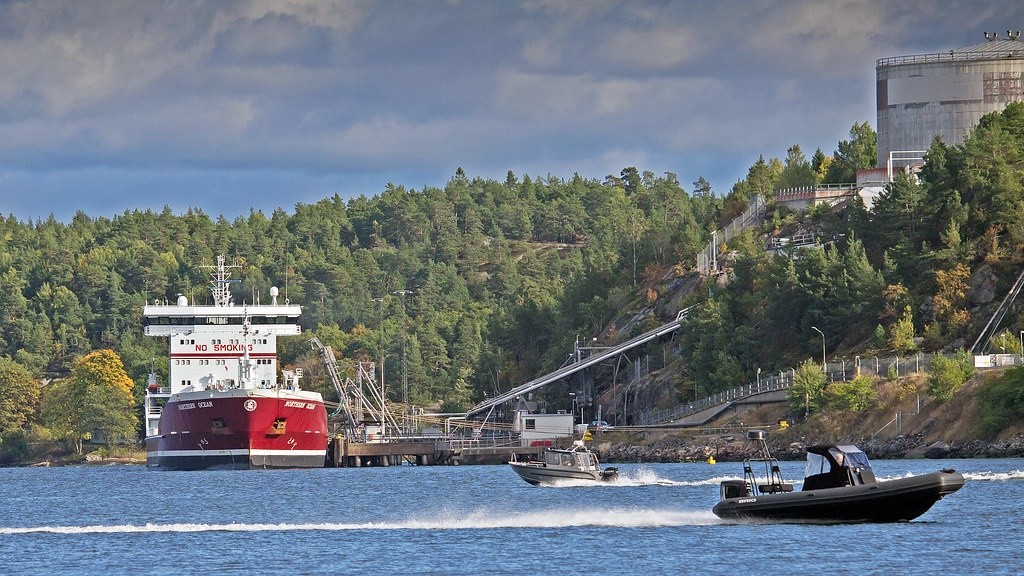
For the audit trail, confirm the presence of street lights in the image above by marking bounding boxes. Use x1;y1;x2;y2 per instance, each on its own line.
812;327;826;375
1021;331;1024;360
757;368;761;385
1001;335;1005;354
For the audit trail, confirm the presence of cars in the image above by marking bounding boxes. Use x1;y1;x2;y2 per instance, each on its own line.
587;421;614;434
574;424;588;432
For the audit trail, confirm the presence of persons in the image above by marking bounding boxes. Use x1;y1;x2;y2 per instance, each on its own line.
829;453;844;472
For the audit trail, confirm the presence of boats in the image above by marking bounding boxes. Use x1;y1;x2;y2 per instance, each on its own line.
712;429;966;525
508;427;619;486
143;252;328;470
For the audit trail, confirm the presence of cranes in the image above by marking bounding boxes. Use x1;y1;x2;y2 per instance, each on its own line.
372;297;385;390
394;290;412;426
308;336;360;439
